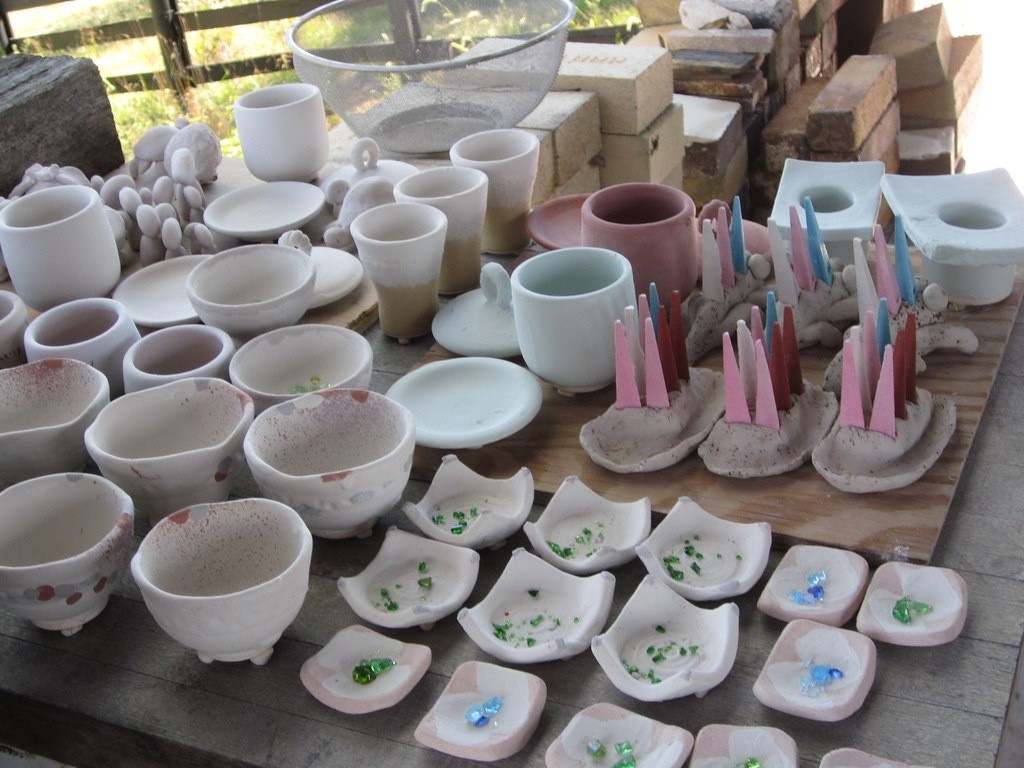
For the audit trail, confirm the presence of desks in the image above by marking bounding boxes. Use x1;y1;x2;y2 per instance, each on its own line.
0;157;1024;768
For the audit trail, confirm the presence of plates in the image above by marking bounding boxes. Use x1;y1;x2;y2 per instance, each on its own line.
384;355;543;450
202;180;326;241
299;453;968;768
307;244;363;311
111;253;215;327
525;193;592;249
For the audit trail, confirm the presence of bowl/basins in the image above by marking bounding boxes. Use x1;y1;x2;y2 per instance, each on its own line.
285;0;576;159
0;322;417;664
187;242;317;346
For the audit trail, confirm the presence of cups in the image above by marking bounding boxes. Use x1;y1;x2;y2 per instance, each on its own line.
23;296;142;393
392;165;489;296
350;201;448;340
234;83;329;182
121;323;235;394
0;184;122;310
510;247;638;392
449;128;540;255
579;181;698;305
0;289;29;368
